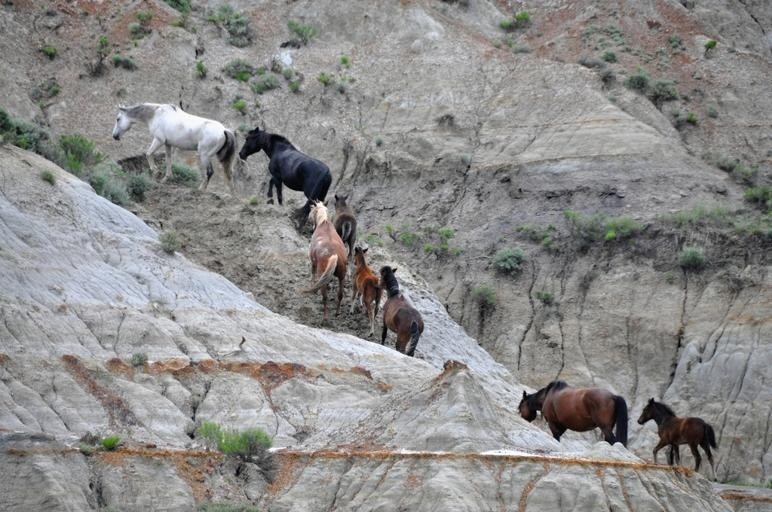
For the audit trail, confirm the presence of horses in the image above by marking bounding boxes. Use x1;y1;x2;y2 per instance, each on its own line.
333;194;357;259
349;247;383;337
638;398;719;482
303;196;347;321
379;267;424;357
239;127;332;229
518;381;628;448
113;102;236;193
